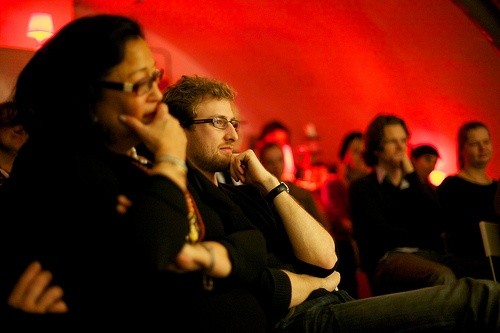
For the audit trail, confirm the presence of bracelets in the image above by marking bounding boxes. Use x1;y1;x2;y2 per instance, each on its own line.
156;155;187;172
267;182;289;202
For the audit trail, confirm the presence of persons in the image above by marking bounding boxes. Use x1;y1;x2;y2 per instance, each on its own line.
248;120;442;301
348;115;458;296
0;14;268;333
0;101;30;187
157;75;500;333
438;121;500;283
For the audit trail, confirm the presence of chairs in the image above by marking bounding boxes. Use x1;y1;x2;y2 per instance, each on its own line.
479;221;500;283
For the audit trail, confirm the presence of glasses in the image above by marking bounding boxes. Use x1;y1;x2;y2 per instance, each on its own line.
191;117;239;132
103;69;163;96
